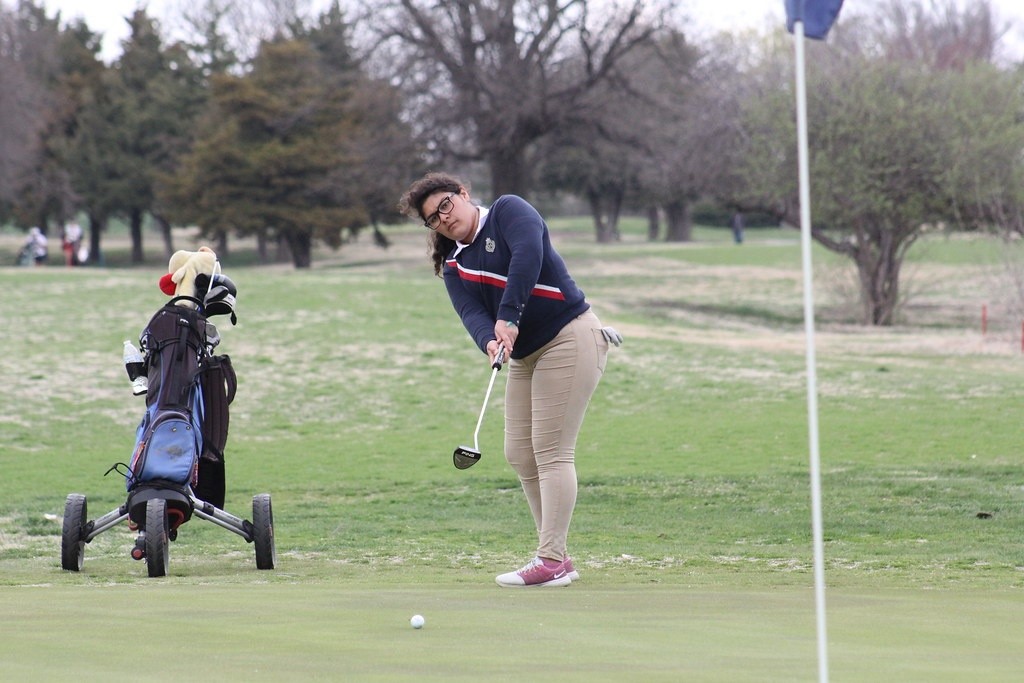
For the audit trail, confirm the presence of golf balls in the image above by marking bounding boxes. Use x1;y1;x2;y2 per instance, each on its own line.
411;614;424;629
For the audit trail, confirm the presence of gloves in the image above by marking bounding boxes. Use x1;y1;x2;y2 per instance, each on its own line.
601;327;624;347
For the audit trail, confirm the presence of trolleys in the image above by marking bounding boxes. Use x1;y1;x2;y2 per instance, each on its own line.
62;277;268;579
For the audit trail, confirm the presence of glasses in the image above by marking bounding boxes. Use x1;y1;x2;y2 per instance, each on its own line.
424;192;456;230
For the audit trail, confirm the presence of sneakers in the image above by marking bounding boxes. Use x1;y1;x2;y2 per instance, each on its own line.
495;556;580;589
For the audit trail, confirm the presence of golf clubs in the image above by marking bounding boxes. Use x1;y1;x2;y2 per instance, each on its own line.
453;340;506;470
194;272;237;324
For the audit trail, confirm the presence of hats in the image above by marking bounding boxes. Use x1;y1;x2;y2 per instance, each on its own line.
199;273;237;325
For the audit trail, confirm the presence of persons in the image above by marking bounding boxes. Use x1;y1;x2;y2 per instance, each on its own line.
28;217;84;266
733;212;744;243
397;171;623;591
